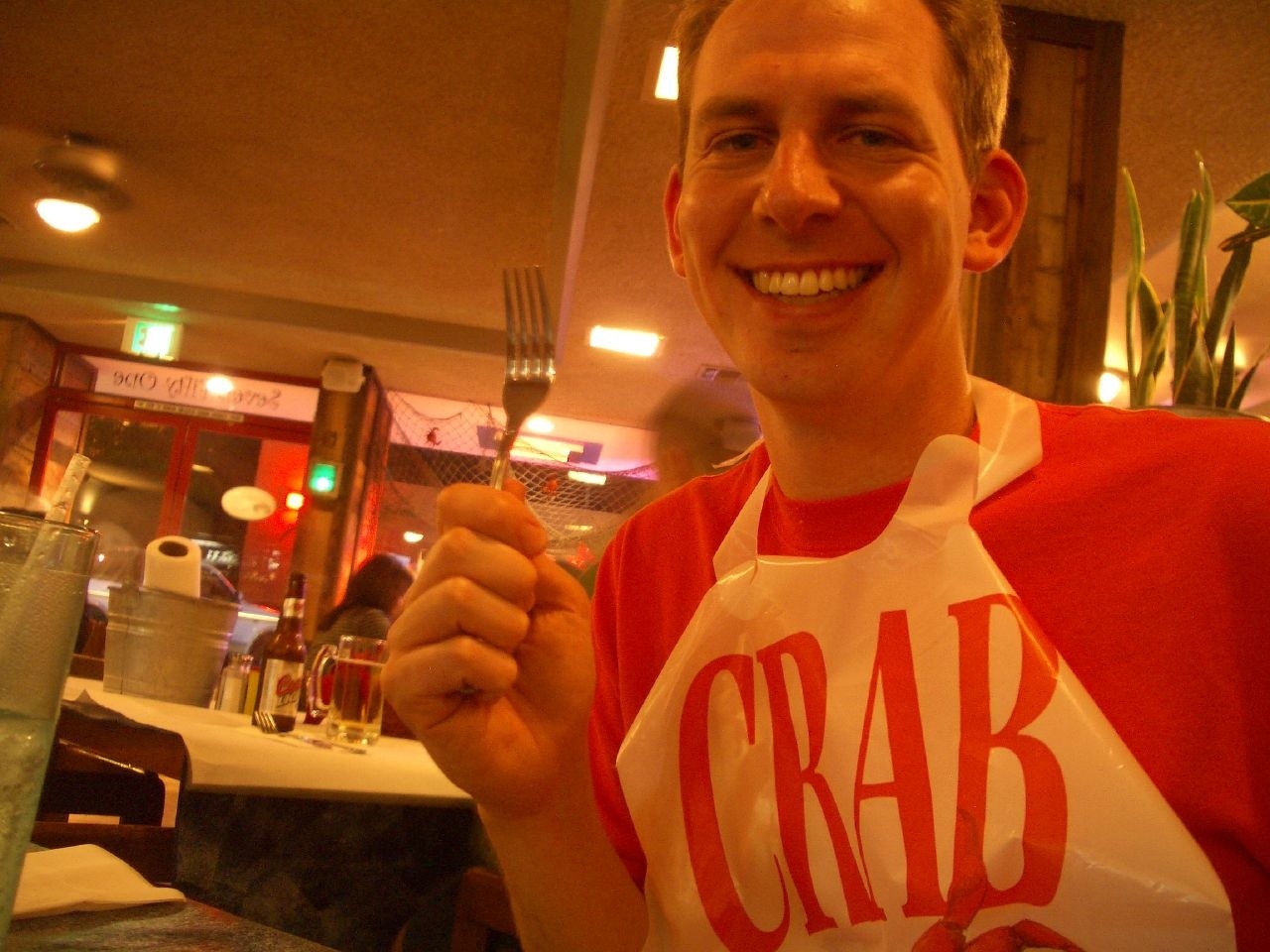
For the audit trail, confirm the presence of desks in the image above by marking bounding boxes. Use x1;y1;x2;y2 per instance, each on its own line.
0;676;477;952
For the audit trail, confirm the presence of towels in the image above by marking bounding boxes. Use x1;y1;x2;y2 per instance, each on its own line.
12;842;189;922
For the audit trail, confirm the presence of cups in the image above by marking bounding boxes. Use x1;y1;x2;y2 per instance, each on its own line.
307;635;390;746
0;509;101;948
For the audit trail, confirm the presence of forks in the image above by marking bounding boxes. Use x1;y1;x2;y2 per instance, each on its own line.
454;264;556;696
254;711;332;749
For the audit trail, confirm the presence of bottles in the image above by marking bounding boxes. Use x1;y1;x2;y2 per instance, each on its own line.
214;651;253;714
251;571;307;732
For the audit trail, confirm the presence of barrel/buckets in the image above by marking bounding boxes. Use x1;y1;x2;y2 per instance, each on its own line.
103;579;244;709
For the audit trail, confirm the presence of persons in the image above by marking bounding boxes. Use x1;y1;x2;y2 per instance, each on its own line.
381;0;1270;952
297;551;416;713
